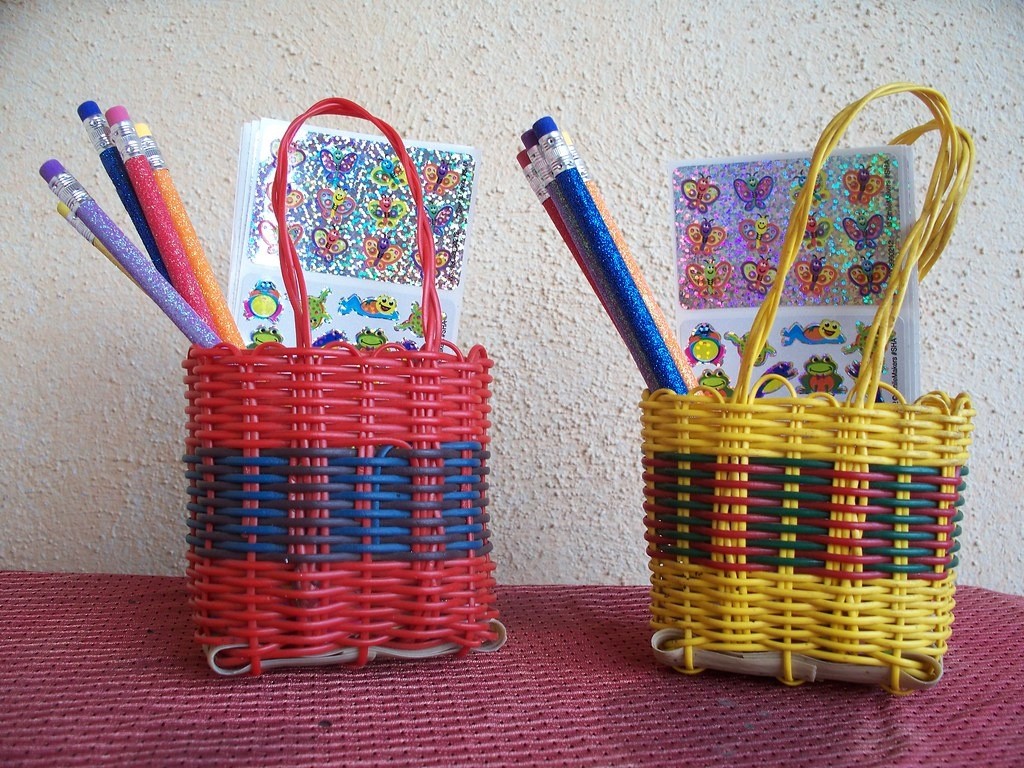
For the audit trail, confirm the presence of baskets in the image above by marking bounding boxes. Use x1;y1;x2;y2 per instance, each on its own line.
639;82;978;696
181;98;506;677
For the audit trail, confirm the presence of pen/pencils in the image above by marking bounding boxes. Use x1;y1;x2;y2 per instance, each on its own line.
515;116;704;394
38;96;246;349
